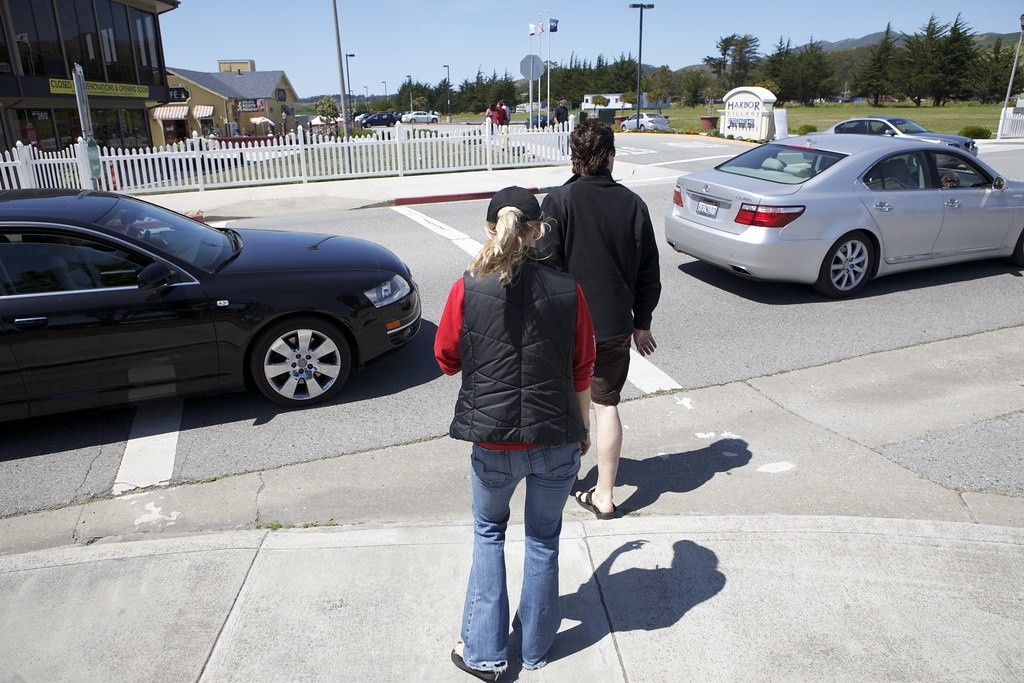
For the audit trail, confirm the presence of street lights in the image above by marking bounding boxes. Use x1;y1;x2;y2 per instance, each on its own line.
346;54;354;120
382;81;387;96
628;3;655;130
406;75;413;113
1004;14;1024;108
443;65;452;123
364;86;368;112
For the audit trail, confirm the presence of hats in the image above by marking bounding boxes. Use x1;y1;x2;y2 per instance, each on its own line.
486;186;543;223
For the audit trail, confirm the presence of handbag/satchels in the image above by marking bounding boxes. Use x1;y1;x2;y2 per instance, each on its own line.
487;108;492;116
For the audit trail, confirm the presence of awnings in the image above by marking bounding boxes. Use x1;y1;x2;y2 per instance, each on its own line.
194;105;213;118
153;106;189;120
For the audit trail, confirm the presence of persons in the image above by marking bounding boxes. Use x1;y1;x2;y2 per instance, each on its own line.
537;119;661;520
485;100;510;125
553;96;569;154
434;180;596;683
50;226;144;290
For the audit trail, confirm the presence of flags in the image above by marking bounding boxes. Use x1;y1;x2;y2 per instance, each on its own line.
529;23;535;35
550;19;558;32
539;23;544;33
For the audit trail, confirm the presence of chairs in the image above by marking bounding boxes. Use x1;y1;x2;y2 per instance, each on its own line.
0;234;64;294
889;159;918;190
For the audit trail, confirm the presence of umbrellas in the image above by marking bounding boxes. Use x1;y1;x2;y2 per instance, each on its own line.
250;117;275;136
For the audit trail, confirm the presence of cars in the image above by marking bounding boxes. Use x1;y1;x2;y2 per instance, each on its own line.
803;116;978;168
348;111;404;123
360;113;397;129
0;188;422;424
401;111;440;124
665;133;1024;302
525;115;553;129
880;96;900;102
620;113;667;132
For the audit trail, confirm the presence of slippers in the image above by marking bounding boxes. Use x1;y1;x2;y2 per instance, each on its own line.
576;489;618;520
451;641;501;683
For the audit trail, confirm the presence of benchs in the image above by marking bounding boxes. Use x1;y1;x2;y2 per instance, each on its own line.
761;158;808;178
